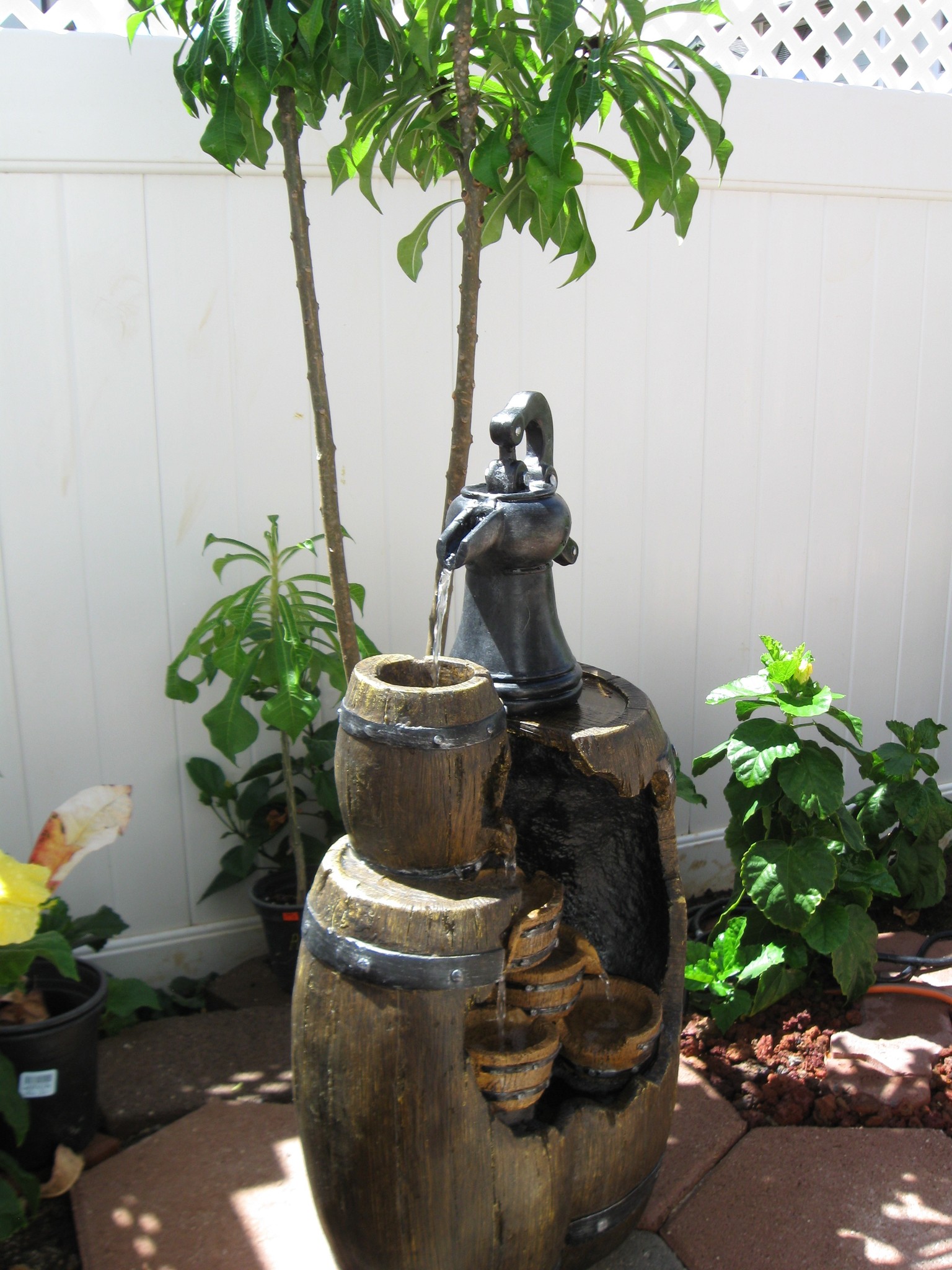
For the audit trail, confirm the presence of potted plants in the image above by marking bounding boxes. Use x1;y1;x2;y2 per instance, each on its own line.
163;511;389;994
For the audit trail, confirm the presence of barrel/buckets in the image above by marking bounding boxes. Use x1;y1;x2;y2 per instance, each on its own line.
289;650;689;1270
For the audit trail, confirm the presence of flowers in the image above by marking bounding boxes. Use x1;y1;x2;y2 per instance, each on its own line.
1;782;141;1023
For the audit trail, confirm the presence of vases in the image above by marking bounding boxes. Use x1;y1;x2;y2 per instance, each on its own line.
0;962;110;1156
